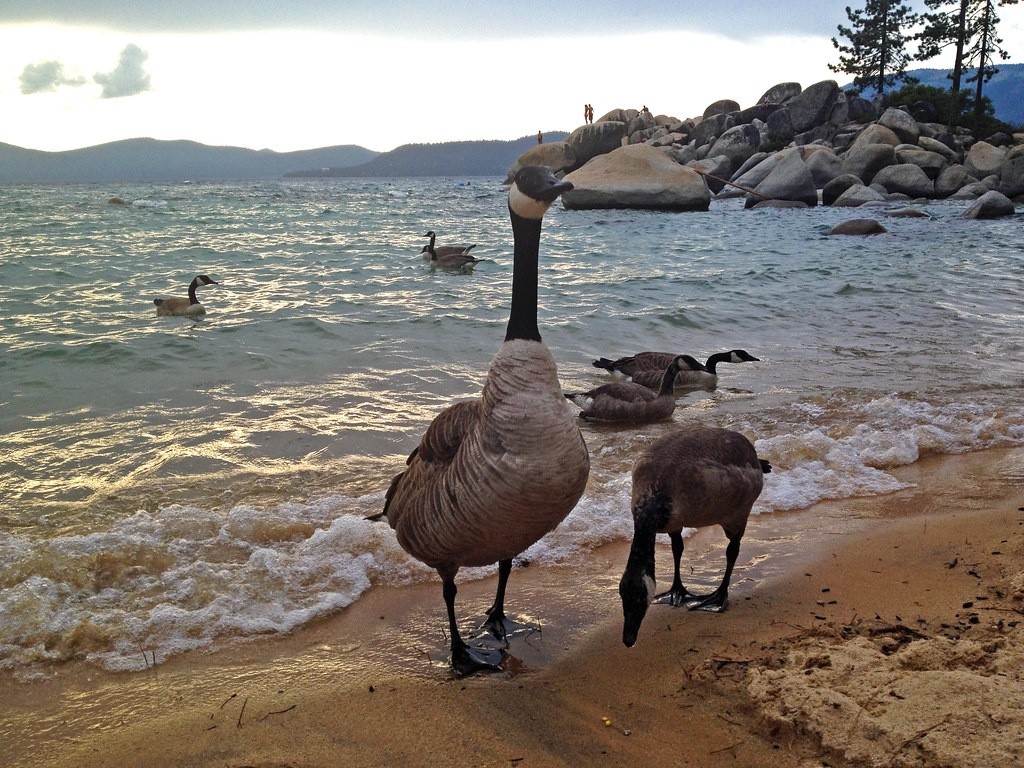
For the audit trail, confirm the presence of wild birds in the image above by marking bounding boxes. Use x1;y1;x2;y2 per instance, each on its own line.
420;245;485;268
592;350;760;388
154;275;219;315
420;231;477;261
563;355;705;421
363;166;591;679
619;428;773;648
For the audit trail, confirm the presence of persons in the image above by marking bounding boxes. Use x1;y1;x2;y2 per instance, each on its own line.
584;104;594;124
640;105;649;112
537;131;543;144
